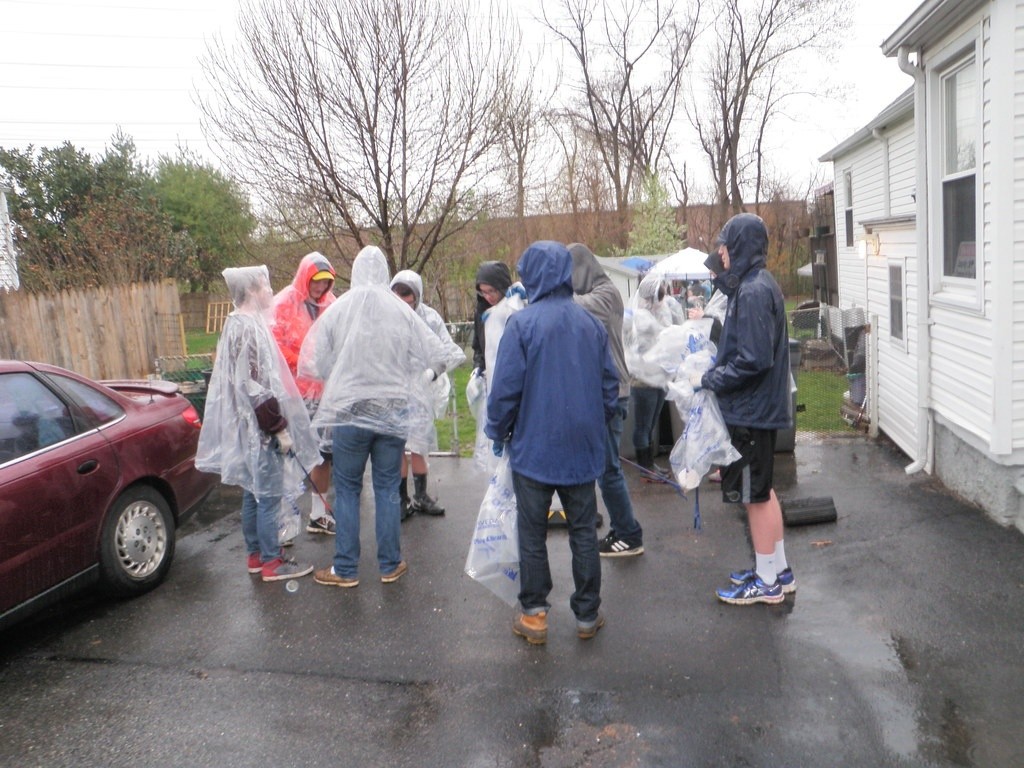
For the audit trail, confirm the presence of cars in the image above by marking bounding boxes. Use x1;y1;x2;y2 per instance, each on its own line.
0;357;220;631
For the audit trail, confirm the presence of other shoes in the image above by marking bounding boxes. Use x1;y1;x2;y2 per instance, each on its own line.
708;469;722;482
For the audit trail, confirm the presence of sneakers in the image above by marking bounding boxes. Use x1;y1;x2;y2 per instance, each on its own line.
597;530;644;557
262;547;314;581
577;609;604;638
248;548;295;573
715;573;785;604
306;512;336;534
313;566;359;587
513;612;548;644
381;561;407;582
729;566;796;593
400;497;413;520
412;495;444;514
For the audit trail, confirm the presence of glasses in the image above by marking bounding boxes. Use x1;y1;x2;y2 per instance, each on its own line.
477;287;498;297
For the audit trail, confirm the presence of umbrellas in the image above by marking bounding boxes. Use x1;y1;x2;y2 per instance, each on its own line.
655;246;719;318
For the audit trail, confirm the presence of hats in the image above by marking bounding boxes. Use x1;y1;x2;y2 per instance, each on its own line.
311;270;334;281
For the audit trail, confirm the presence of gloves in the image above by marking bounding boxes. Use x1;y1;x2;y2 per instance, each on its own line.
275;429;296;458
505;281;526;300
493;440;503;456
689;371;704;392
470;367;480;376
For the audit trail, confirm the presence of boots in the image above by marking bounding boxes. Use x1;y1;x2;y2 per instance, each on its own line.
636;441;671;483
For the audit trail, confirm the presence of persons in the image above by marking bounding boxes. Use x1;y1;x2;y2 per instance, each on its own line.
298;247;446;587
562;244;643;556
388;270;466;521
268;253;341;548
472;261;511;380
703;252;737;481
701;214;799;604
485;240;616;615
631;276;672;481
193;265;324;581
